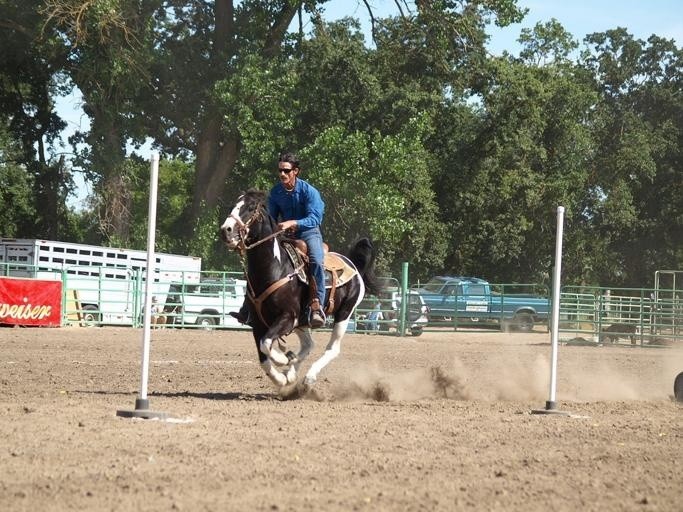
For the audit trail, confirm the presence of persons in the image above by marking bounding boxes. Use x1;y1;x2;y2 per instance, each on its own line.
597;290;611;317
228;153;328;331
137;294;167;331
363;304;383;335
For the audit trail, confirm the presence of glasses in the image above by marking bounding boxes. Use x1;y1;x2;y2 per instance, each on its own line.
277;164;296;174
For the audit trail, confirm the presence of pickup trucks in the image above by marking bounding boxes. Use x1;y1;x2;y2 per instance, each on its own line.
410;275;553;332
163;278;254;331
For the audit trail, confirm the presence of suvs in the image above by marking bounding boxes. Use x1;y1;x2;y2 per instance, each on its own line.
349;287;430;336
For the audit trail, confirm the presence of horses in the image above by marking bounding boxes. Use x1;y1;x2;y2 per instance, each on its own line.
216;187;388;401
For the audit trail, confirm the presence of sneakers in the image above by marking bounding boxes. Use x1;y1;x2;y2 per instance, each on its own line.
310;311;324;328
230;311;252;326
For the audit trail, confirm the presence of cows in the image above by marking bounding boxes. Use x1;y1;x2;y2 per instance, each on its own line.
600;322;639;348
